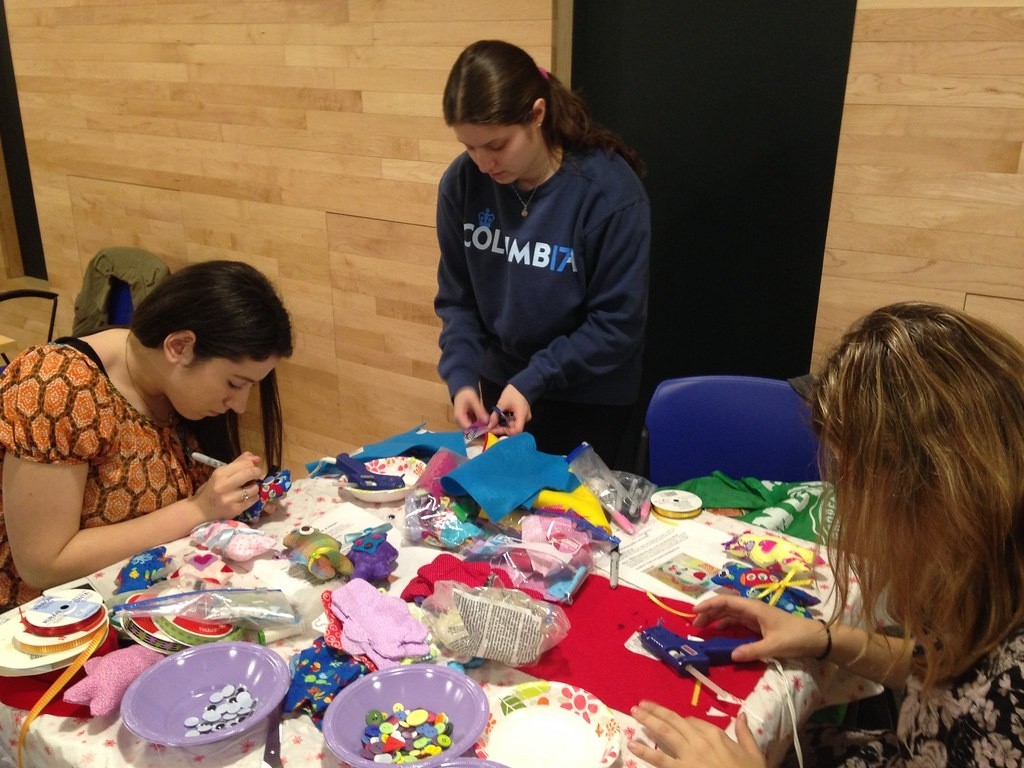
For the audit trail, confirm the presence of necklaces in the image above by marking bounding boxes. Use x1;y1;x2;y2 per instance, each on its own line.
126;332;175;424
511;165;547;216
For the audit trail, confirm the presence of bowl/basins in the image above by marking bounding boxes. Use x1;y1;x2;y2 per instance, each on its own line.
340;456;427;502
118;639;291;747
321;663;622;768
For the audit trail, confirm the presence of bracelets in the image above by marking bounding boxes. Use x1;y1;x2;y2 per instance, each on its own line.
815;619;832;660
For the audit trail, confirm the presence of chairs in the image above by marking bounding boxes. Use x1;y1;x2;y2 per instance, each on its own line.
0;247;171;367
634;373;820;486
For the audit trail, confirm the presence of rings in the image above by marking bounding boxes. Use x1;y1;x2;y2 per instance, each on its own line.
242;487;249;502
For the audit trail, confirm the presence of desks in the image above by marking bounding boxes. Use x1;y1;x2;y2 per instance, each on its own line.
1;428;900;768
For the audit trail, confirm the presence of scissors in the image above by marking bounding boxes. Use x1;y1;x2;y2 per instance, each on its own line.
489;406;517;430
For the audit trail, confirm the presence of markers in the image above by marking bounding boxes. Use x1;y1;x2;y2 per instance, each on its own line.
192;452;227;468
601;471;656;535
610;535;620;589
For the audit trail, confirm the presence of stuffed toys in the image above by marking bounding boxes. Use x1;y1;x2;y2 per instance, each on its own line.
115;546;171;611
63;645;164;717
191;520;276;561
345;523;399;583
712;532;826;620
283;526;353;580
236;469;291;525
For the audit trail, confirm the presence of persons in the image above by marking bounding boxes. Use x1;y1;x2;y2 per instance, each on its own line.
435;40;650;471
0;260;294;615
628;300;1024;768
